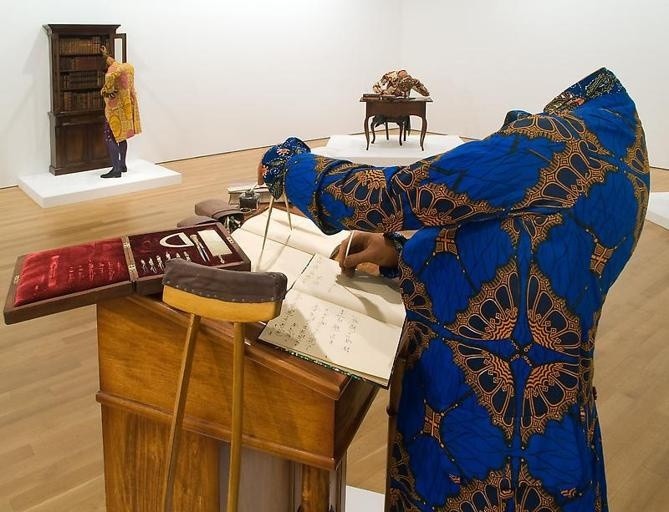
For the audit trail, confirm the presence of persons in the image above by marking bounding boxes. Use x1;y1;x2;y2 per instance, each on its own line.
99;46;142;178
257;67;649;511
372;69;430;136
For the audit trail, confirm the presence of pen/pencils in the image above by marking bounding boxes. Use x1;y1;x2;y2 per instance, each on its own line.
340;229;355;274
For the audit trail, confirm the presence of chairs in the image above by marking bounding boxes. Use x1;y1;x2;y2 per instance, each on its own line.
385;90;410;141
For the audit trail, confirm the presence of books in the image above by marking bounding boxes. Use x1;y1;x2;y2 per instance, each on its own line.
59;37;109;113
256;253;408;391
230;206;352;293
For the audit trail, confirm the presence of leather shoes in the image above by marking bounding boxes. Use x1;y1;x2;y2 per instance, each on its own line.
101;167;126;178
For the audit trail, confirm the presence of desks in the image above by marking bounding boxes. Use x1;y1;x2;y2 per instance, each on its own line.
357;93;432;151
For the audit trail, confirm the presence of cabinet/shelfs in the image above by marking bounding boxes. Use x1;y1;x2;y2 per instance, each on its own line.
42;22;125;178
